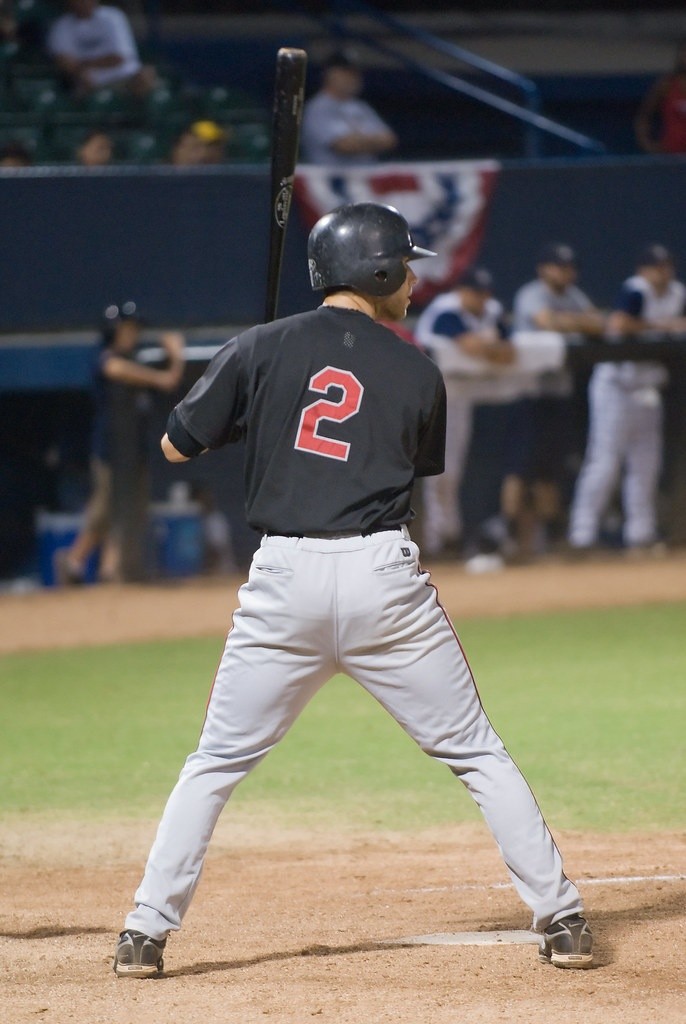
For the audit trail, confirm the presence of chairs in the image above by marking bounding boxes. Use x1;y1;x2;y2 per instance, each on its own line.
0;38;274;168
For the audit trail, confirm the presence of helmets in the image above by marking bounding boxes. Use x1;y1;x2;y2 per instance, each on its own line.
307;200;437;300
101;301;147;346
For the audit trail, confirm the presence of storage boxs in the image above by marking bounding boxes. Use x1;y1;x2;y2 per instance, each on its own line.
35;506;211;588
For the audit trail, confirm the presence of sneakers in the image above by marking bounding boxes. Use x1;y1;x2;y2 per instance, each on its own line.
542;915;598;970
113;929;169;978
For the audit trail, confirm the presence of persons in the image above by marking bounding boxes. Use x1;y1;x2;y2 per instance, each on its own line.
113;200;594;979
79;129;113;164
413;269;514;557
174;120;232;166
568;243;686;550
54;311;184;584
502;241;603;550
298;54;395;163
52;1;159;109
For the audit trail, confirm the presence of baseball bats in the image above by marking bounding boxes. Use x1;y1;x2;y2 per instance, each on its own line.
262;45;309;324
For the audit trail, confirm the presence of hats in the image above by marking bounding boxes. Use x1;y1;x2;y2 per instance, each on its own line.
639;241;677;267
328;48;373;73
191;119;229;143
459;263;497;293
537;242;583;268
